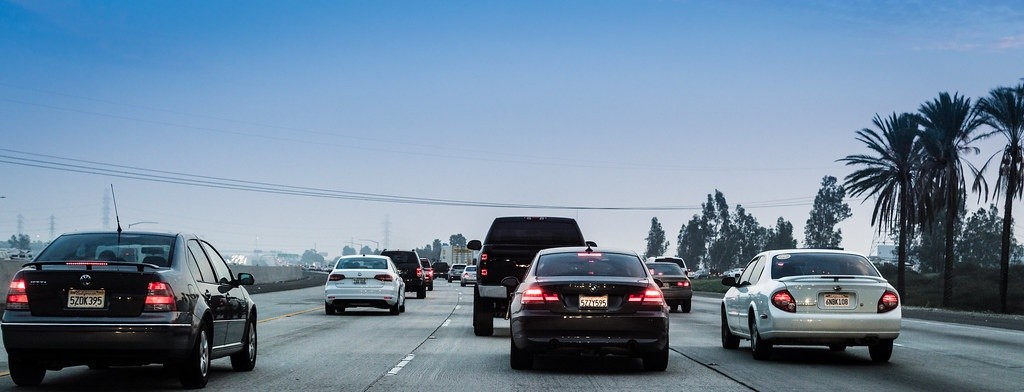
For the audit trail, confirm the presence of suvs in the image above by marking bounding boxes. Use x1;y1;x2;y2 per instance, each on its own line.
380;248;427;299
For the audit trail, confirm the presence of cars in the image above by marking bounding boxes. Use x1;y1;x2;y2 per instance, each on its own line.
501;242;672;373
721;247;903;364
644;262;692;312
645;256;688;277
0;248;38;261
225;253;334;272
0;236;259;391
688;266;745;278
461;265;477;286
324;255;406;316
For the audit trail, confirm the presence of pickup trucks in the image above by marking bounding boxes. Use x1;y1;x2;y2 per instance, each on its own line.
420;257;433;291
432;262;450;280
447;264;467;283
466;215;599;336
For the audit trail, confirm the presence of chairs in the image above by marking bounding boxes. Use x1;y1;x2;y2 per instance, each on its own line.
344;262;360;268
142;256;168;267
373;262;384;268
98;250;127;262
